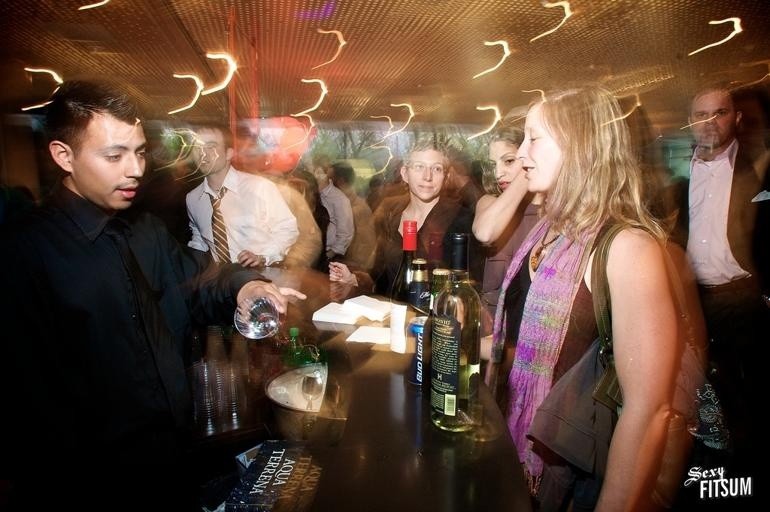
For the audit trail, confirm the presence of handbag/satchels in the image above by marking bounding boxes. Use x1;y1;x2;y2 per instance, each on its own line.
526;223;712;483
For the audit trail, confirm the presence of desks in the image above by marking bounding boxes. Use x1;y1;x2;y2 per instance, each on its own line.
221;262;534;512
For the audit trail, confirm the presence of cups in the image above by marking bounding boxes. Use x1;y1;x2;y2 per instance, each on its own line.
233;296;281;339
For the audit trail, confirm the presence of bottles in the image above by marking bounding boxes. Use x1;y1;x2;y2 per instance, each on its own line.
389;219;418;301
281;326;303;371
405;257;434;387
430;232;482;432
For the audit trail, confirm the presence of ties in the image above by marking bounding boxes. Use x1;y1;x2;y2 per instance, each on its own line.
205;186;232;266
102;216;193;424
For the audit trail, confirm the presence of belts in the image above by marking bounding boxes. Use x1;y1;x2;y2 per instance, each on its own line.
695;269;766;297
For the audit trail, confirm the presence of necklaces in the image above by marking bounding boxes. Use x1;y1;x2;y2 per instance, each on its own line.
528;221;560;271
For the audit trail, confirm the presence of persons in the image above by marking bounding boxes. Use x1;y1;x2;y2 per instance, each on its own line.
473;127;548;315
486;91;707;510
445;146;482;209
0;79;309;512
308;158;355;272
366;163;408;212
181;123;297;268
652;84;770;295
237;143;322;273
329;143;477;304
289;170;328;273
329;161;375;270
